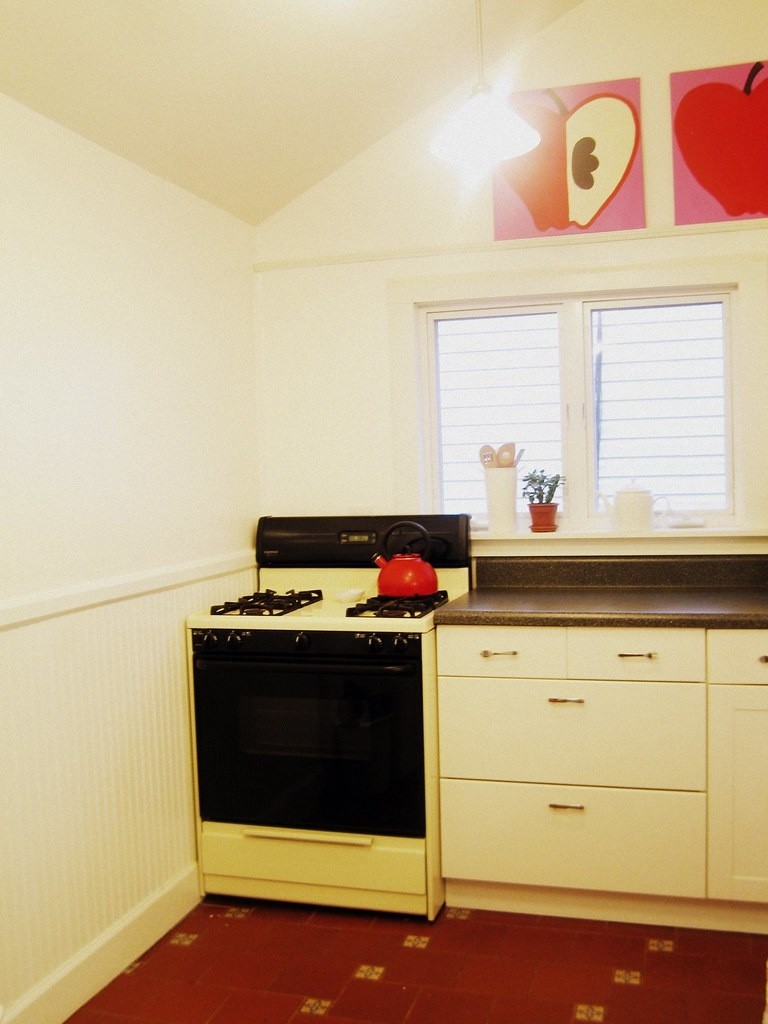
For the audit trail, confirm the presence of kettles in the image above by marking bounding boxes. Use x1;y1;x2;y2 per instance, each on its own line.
369;520;441;599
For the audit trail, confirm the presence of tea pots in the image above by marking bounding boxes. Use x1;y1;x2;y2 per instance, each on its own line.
599;488;672;533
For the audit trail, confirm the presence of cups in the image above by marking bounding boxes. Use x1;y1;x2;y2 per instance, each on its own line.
485;466;517;533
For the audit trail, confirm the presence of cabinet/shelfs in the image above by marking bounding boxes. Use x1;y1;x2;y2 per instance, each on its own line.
195;819;433;923
433;611;708;933
706;612;767;935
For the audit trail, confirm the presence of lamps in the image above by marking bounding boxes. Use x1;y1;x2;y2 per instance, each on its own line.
433;1;542;165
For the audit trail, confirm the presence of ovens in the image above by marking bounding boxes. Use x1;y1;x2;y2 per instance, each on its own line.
185;626;442;924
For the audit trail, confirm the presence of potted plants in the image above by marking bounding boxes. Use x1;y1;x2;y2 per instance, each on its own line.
522;469;566;532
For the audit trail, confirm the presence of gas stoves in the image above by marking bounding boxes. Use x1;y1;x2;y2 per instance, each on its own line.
184;511;472;635
